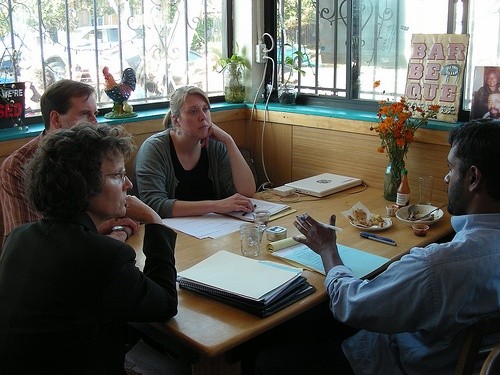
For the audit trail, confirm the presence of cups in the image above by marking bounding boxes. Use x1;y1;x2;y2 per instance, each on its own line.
240;223;259;257
246;226;264;247
411;223;429;236
251;210;271;232
385;204;400;217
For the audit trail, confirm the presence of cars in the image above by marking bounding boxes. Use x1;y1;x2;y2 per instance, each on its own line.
276;42;311;66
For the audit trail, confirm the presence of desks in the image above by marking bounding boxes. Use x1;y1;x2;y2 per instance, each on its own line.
123;184;454;375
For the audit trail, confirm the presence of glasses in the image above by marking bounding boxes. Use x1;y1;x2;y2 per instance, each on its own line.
101;169;127;181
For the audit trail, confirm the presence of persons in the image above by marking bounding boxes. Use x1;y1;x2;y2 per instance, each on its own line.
0;79;141;249
131;85;256;219
470;70;500;120
292;117;500;375
0;120;179;375
482;91;500;120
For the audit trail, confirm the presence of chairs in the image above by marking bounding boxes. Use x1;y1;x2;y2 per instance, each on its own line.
454;315;500;375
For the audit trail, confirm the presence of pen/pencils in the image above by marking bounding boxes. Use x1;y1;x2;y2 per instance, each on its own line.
296;216;343;232
242;204;256;216
360;232;395;243
360;234;397;246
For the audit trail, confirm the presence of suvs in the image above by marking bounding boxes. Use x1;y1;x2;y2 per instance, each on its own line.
34;25;119;76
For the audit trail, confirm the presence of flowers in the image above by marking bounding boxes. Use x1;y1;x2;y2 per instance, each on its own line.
369;80;441;200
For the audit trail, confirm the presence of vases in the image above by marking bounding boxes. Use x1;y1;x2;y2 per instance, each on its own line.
223;62;246;103
277;63;298;105
384;160;406;202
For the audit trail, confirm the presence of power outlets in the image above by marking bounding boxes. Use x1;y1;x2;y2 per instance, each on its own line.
255;43;268;63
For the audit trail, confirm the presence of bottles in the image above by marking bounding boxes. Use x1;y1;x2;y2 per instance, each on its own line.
398;169;410;210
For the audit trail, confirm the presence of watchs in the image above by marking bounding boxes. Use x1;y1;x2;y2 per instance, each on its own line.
112;225;129;241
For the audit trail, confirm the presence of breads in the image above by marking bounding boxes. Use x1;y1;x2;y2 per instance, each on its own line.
347;210;385;226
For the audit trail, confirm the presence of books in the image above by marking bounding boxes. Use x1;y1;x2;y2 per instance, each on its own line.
176;249;317;318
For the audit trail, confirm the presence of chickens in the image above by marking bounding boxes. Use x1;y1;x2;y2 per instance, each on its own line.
102;66;136;103
121;101;133;113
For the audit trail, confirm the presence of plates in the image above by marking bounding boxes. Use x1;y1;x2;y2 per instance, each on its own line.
348;216;392;232
395;205;445;226
